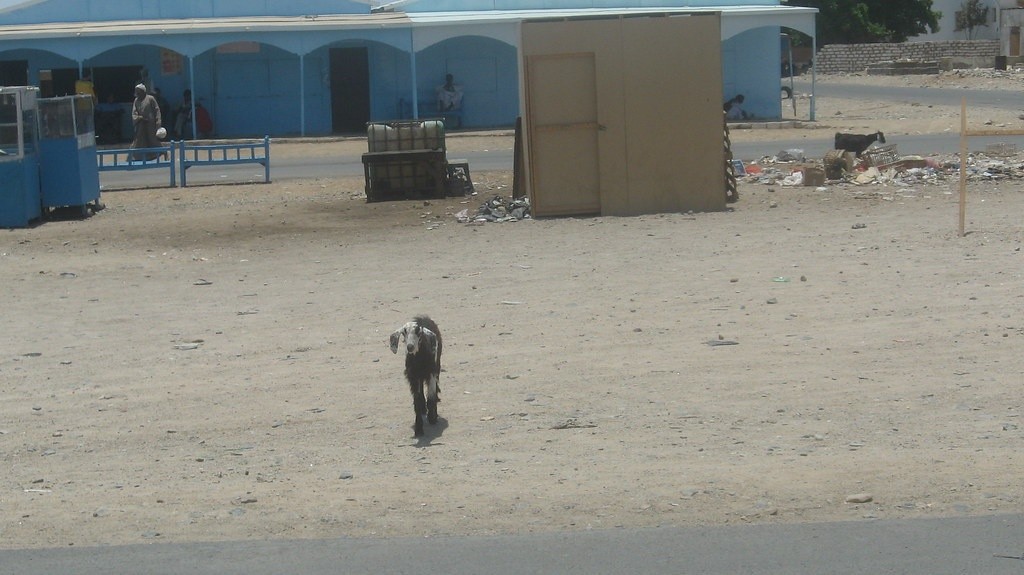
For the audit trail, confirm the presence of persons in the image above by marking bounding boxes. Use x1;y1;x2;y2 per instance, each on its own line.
151;87;213;139
94;92;125;145
124;83;169;163
74;68;97;135
436;74;463;113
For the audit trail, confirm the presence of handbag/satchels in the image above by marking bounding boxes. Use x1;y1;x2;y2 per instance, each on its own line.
156;126;167;139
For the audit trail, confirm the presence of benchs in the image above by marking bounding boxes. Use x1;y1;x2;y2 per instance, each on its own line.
402;93;465;131
96;140;175;188
179;134;271;186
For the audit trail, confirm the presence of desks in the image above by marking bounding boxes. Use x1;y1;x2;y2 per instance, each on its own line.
362;148;447;204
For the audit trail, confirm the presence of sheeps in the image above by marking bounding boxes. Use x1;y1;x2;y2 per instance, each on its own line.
834;130;886;157
389;316;442;435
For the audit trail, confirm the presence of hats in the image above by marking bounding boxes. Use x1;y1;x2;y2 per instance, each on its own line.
135;84;147;92
